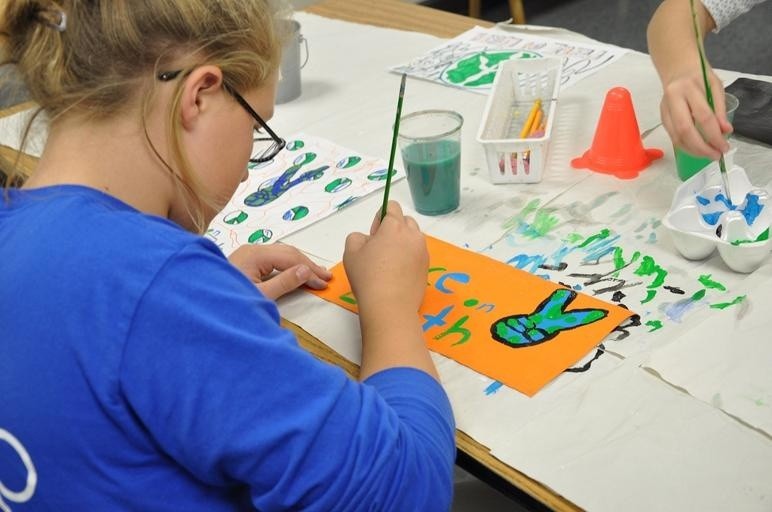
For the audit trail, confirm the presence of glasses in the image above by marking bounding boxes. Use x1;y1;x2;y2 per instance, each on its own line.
157;61;287;163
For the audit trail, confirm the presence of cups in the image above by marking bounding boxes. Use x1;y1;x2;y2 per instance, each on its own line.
392;109;463;217
671;92;738;182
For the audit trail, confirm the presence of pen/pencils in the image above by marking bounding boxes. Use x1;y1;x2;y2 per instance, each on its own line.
499;100;547;172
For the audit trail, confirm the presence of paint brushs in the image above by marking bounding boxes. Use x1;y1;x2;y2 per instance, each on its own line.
380;72;407;223
691;1;732;205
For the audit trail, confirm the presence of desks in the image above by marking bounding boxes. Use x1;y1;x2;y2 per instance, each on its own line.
2;0;772;512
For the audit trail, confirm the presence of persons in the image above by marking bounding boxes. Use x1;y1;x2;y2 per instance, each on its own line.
646;0;766;162
0;0;454;512
